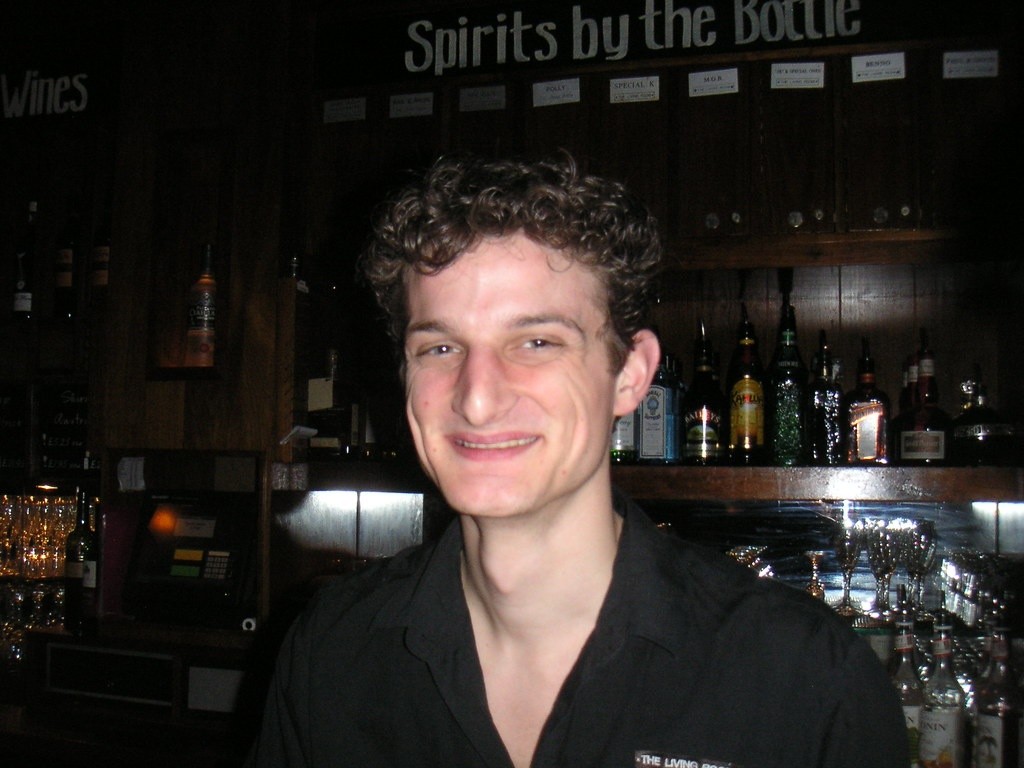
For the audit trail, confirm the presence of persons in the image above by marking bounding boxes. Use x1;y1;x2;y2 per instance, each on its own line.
243;149;910;768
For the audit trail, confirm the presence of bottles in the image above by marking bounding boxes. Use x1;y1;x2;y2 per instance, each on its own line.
1;228;1024;768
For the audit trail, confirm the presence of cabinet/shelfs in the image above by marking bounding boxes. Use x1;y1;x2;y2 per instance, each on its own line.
302;34;1024;302
